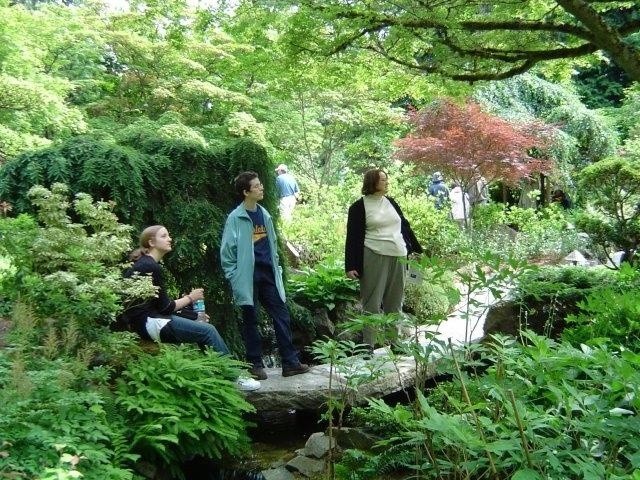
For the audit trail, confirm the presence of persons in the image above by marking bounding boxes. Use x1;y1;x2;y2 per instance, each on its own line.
468;171;488;208
345;169;423;359
220;173;311;379
553;190;576;210
427;171;452;220
276;164;300;225
448;179;469;228
125;225;261;391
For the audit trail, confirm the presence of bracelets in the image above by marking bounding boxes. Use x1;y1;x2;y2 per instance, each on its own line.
186;295;192;304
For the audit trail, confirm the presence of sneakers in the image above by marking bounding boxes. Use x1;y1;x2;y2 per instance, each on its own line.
282;362;308;377
236;369;267;390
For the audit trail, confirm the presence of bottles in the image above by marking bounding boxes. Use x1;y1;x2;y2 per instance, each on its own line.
192;294;205;323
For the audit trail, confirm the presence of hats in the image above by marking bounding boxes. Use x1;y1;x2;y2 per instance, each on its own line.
433;172;443;182
275;164;287;171
